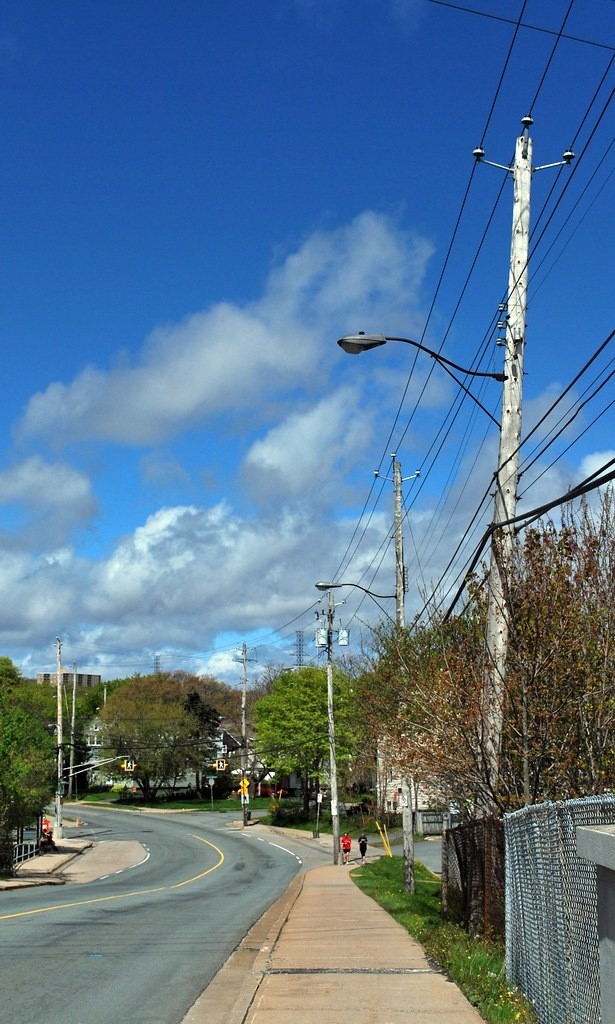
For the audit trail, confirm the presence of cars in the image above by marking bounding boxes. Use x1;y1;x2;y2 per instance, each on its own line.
256;783;288;799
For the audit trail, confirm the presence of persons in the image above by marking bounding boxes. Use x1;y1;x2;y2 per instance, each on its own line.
342;831;351;865
359;832;368;866
243;805;248;826
38;829;55;856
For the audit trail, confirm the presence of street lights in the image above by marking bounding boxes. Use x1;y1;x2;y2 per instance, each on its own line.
337;332;528;932
314;580;417;894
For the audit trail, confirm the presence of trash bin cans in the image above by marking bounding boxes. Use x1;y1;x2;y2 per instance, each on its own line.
247;811;251;820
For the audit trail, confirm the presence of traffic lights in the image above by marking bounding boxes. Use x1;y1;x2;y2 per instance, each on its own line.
215;759;226;771
124;759;134;771
